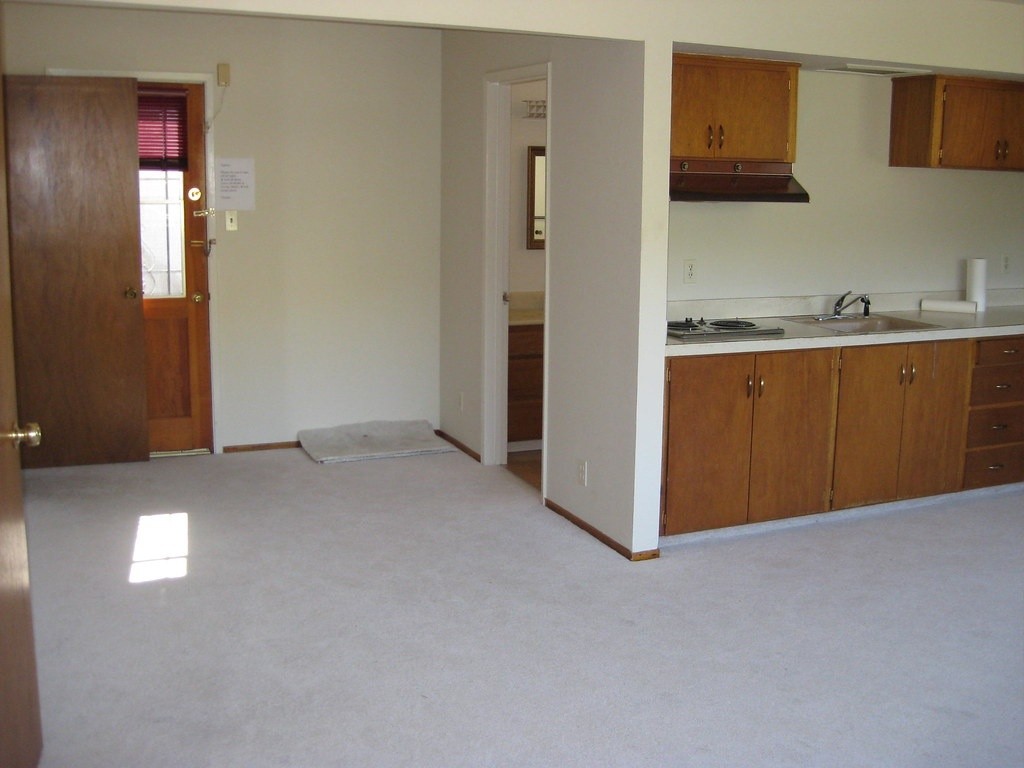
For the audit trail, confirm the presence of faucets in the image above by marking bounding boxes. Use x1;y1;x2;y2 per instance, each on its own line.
833;291;867;315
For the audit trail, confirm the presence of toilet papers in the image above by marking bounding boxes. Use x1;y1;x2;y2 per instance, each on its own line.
919;258;988;313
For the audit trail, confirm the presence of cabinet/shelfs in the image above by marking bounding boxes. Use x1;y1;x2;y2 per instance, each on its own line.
961;335;1024;490
832;338;975;512
888;74;1024;174
670;52;802;176
657;348;843;536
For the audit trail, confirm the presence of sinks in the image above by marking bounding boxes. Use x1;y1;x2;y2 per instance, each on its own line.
802;317;947;335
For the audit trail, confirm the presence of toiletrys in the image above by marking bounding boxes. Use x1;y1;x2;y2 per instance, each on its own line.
863;294;870;316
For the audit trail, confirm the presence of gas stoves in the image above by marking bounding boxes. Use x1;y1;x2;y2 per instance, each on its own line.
667;318;784;340
